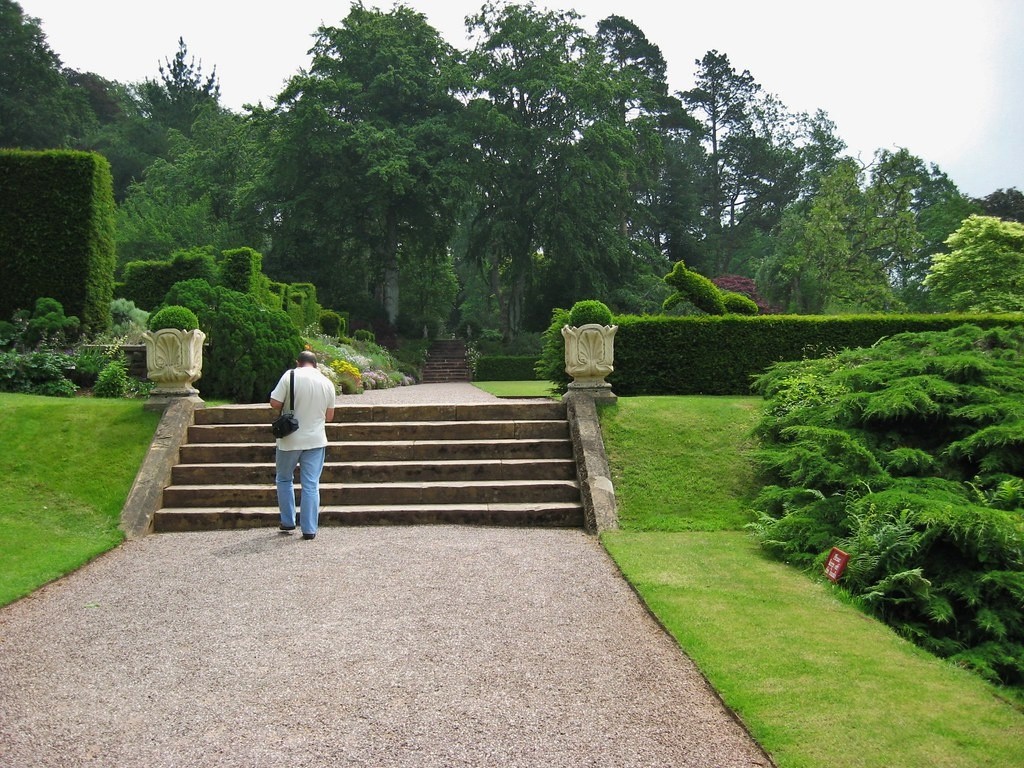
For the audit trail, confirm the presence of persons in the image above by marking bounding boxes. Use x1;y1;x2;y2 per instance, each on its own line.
270;351;335;539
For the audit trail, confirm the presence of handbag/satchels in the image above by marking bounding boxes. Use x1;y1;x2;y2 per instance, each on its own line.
272;370;299;438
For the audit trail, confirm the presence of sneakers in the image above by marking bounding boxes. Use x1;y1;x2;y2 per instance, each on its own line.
277;524;295;535
303;533;316;539
